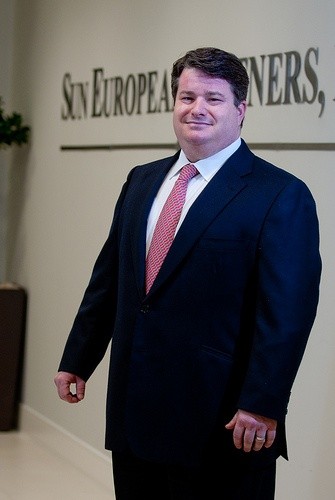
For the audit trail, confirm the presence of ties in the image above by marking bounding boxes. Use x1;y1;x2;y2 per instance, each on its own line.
145;165;199;292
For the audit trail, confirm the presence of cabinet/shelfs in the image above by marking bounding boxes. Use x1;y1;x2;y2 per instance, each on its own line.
0;280;27;430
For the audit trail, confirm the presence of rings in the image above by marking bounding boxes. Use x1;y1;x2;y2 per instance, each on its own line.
255;435;266;442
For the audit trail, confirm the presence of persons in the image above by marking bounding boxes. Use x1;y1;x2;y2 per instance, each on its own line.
52;51;325;500
